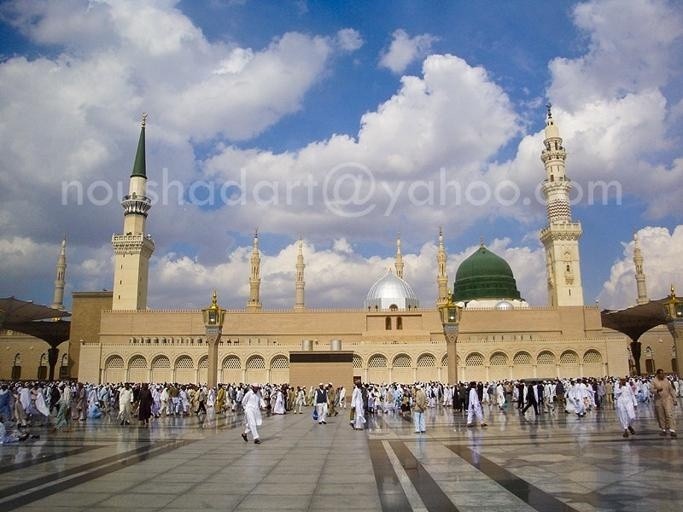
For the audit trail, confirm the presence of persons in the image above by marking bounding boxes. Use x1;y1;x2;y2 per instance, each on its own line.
649;369;678;436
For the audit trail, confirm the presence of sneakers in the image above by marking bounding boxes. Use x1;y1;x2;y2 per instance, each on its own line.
468;424;474;427
481;424;488;427
319;421;326;425
671;432;678;438
240;434;248;442
254;439;261;444
120;420;129;425
350;423;355;429
659;431;666;436
623;433;629;437
628;426;636;434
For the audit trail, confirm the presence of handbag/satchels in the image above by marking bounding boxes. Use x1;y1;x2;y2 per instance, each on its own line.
312;410;318;420
